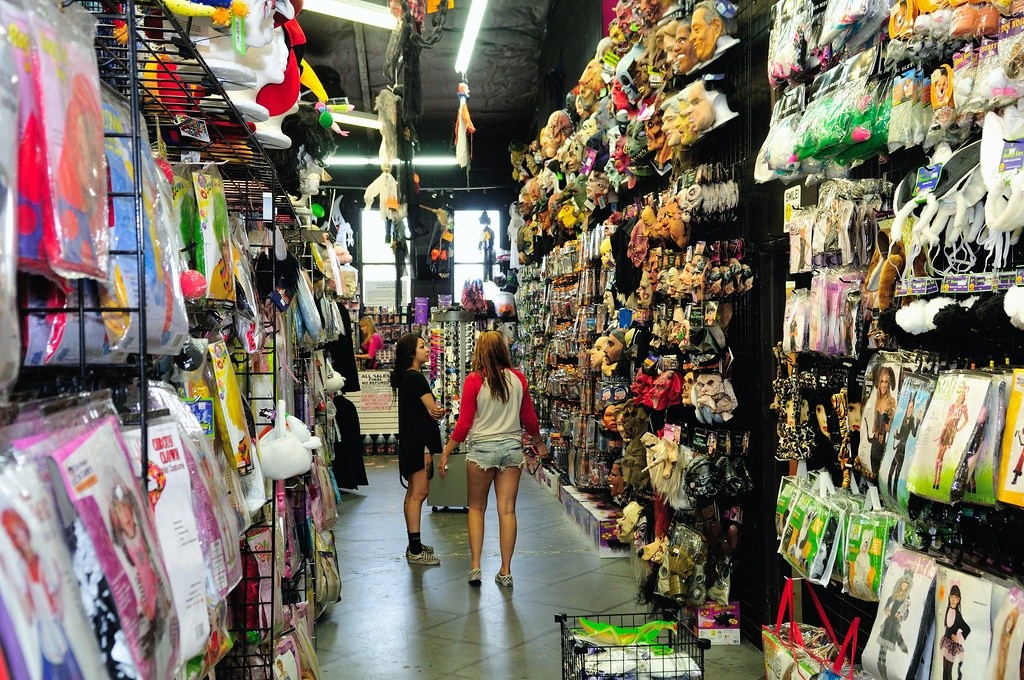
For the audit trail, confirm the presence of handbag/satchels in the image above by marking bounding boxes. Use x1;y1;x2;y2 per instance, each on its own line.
762;578;848;680
817;617;875;680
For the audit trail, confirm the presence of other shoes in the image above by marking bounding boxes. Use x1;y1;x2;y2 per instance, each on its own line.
467;569;481;582
495;572;513;587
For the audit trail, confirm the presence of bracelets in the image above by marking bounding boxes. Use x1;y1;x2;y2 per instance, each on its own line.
536;440;543;444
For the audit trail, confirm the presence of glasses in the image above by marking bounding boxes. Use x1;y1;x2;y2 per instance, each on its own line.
429;321;477;454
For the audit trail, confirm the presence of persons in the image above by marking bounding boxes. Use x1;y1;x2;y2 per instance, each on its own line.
865;367;895;473
851;530;871;598
878;570;914;680
933;384;969;490
790;509;819;559
888;392;923;502
994;609;1019;680
453;83;474;169
389;335;445;566
105;470;174;654
354;316;384;370
590;328;628;376
0;507;82;680
364;161;397;220
940;585;970;680
673;0;740;133
288;146;324;207
177;0;302;149
479;211;495;281
439;331;549;587
783;498;795;528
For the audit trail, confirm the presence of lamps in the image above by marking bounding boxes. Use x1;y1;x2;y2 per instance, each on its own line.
301;0;489;166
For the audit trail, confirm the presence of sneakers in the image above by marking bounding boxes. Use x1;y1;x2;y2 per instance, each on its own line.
408;550;440;565
406;544;433;557
